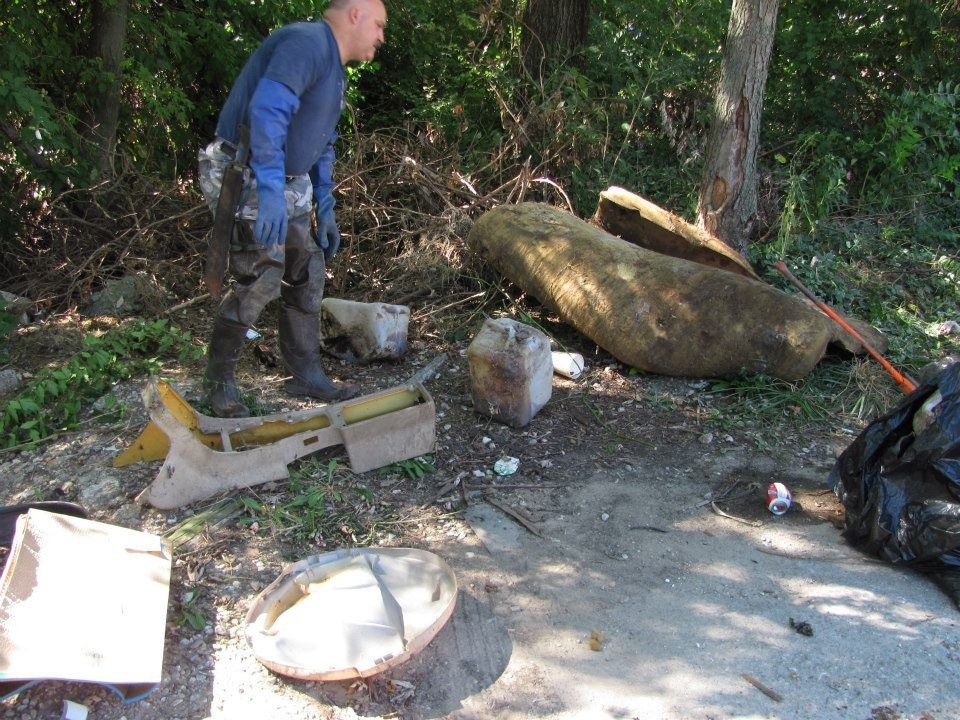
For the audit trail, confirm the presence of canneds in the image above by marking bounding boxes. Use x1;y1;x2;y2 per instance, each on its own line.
766;482;792;515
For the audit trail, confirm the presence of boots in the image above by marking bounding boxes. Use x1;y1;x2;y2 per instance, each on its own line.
277;303;355;400
202;315;251;418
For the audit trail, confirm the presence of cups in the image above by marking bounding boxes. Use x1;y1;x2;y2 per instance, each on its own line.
550;350;585;380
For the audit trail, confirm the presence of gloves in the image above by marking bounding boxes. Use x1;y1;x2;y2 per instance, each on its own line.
248;78;299;246
308;130;341;259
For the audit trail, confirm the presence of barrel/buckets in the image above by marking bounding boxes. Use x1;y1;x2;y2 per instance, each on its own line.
322;297;410;362
466;315;553;428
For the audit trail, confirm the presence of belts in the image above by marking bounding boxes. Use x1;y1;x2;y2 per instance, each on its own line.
219;143;238;161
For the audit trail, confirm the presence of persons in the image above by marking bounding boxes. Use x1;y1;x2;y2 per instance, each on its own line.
197;0;388;418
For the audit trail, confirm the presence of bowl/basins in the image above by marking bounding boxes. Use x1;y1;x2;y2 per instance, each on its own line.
1;501;90;548
